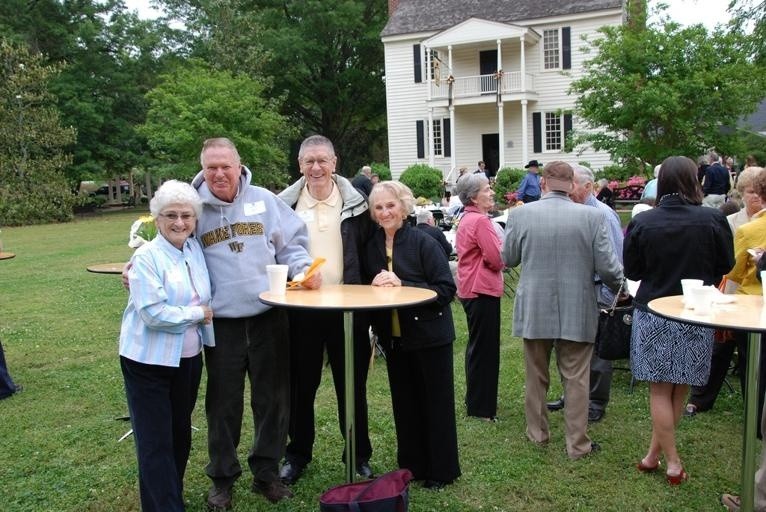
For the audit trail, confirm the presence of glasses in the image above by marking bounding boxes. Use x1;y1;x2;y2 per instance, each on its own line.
302;156;335;166
159;212;195;219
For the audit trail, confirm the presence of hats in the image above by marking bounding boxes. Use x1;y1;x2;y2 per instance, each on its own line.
524;160;543;169
543;161;574;193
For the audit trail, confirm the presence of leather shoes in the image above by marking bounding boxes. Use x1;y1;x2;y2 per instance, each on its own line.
355;462;373;478
251;480;294;504
584;443;601;458
546;396;564;410
279;462;307;484
587;409;606;423
207;484;232;511
685;404;698;417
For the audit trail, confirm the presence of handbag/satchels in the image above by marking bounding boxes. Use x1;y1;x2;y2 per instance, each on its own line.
319;468;413;512
593;274;636;360
715;276;734;343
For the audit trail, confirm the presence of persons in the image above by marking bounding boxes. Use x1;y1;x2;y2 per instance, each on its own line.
685;168;762;414
699;153;762;196
501;160;623;458
546;166;623;422
351;167;379;195
359;181;461;489
639;164;663;205
455;175;505;418
475;162;489;175
517;159;543;206
623;157;736;486
191;137;322;512
727;167;766;442
118;180;207;511
416;212;451;261
277;137;376;487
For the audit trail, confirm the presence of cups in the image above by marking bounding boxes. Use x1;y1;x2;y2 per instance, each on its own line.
680;279;704;310
691;285;714;320
265;263;289;299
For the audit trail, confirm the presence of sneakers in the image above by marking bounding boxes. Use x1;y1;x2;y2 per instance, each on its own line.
717;493;742;512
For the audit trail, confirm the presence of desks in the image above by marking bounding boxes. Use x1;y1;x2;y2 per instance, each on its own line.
647;291;766;512
86;261;129;422
258;283;438;485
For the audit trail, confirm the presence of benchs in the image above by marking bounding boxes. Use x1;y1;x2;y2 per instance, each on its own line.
610;184;646;210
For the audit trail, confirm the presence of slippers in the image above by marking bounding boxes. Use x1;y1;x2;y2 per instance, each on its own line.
637;461;660;473
668;469;688;486
